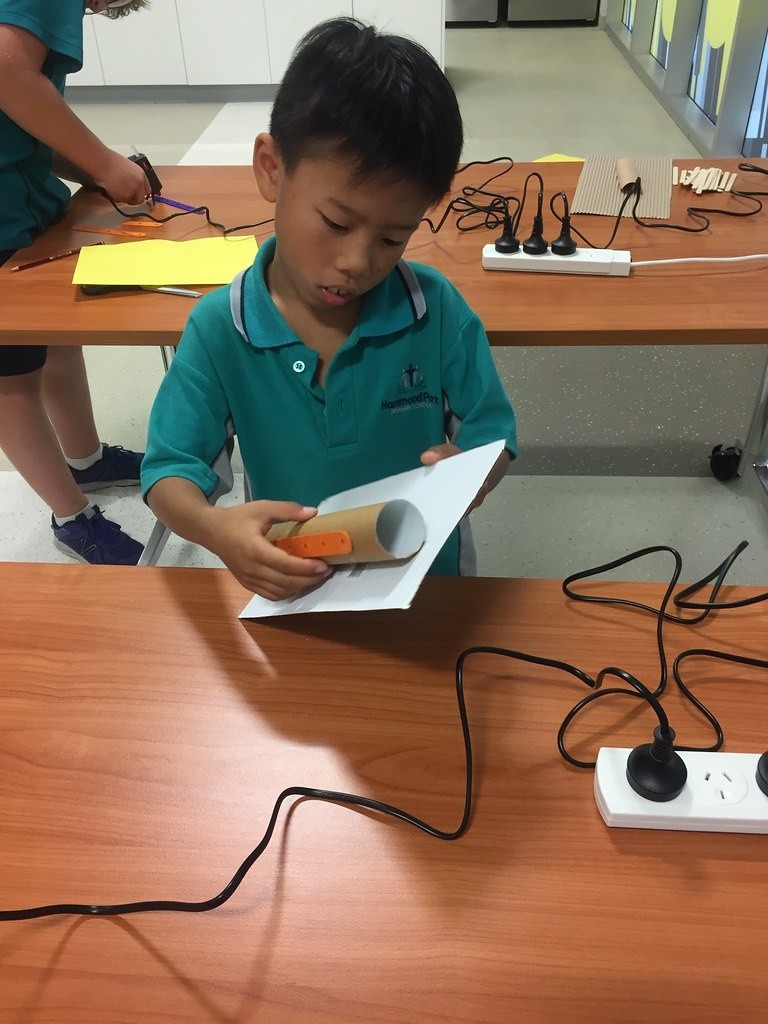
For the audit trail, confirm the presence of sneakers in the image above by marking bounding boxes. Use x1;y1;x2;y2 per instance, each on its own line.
51;504;145;566
65;441;147;493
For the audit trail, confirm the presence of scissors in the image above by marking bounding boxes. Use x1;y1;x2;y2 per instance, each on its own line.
80;284;202;298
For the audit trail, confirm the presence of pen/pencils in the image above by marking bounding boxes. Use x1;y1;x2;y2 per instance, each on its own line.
11;240;105;272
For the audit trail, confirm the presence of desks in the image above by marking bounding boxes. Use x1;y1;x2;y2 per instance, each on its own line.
2;163;768;565
0;555;767;1022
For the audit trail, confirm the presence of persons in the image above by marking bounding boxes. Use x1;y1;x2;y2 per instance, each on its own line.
139;13;519;604
0;0;154;567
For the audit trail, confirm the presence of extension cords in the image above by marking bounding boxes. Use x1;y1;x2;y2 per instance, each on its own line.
593;745;768;835
481;242;633;278
